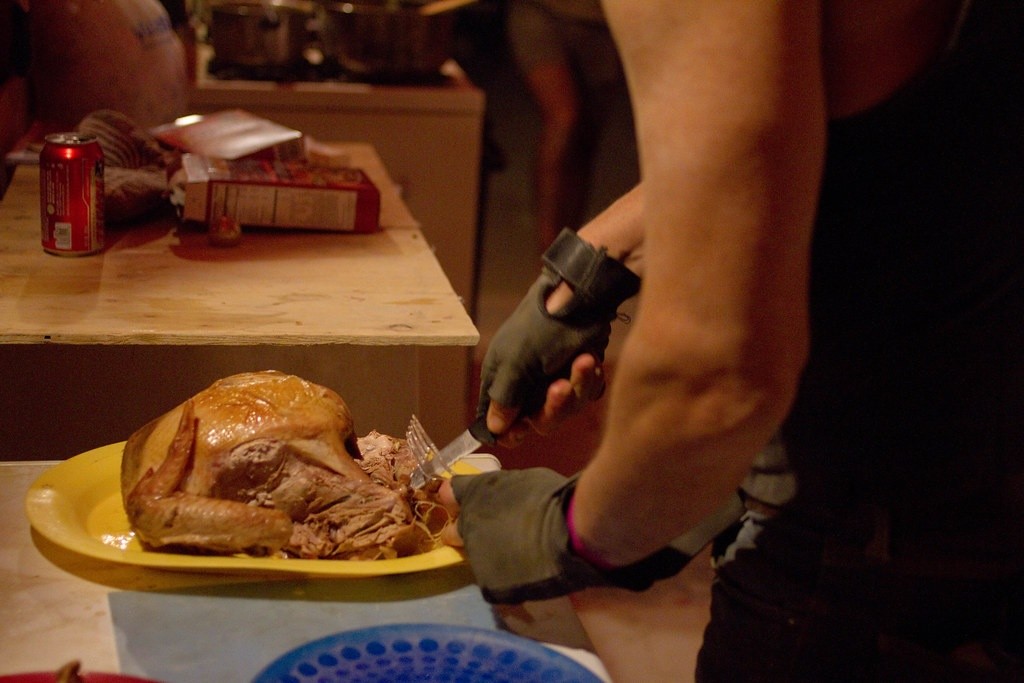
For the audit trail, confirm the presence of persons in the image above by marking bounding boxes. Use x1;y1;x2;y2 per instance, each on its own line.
508;0;642;260
439;0;1024;683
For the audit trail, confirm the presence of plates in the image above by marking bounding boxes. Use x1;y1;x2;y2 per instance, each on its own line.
29;438;470;577
251;623;603;683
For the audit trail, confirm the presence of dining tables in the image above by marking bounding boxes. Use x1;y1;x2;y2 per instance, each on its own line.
1;453;616;683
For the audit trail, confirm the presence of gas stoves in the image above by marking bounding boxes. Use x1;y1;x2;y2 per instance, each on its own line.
174;0;484;118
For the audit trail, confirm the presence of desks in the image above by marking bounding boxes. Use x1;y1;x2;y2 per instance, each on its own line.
0;130;482;439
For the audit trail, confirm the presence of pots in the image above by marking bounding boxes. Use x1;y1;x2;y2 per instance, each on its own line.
207;2;319;80
311;0;455;83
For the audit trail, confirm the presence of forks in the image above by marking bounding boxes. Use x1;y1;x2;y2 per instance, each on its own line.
404;414;461;483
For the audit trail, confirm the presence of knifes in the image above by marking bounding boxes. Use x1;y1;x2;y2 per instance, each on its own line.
400;386;548;490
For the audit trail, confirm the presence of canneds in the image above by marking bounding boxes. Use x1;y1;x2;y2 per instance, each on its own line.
39;131;104;256
